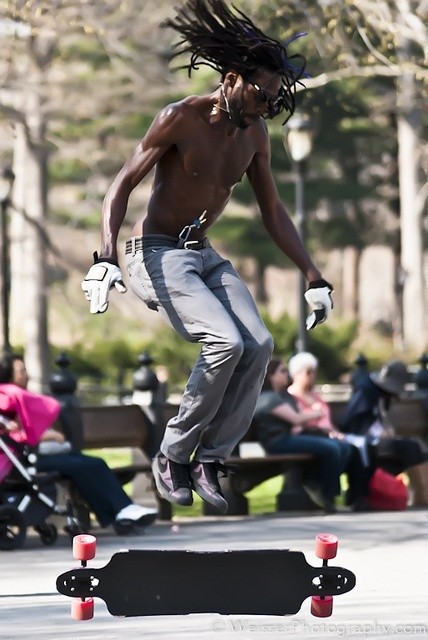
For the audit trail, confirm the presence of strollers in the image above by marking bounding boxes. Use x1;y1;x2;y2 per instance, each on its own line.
0;433;82;550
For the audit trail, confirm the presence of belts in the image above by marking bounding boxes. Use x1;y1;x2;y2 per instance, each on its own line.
125;236;208;255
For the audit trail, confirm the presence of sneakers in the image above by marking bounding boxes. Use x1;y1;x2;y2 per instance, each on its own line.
150;449;193;506
189;459;229;513
113;504;158;534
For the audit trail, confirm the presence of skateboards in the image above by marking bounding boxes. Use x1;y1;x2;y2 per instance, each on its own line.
55;533;356;621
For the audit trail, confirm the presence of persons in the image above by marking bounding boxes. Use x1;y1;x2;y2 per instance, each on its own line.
1;354;158;533
255;358;349;509
81;1;333;510
286;350;373;514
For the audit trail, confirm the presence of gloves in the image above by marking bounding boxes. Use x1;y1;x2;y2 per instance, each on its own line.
303;280;334;332
81;251;127;315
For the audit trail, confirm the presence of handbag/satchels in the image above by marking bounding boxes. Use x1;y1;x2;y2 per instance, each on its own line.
367;466;408;511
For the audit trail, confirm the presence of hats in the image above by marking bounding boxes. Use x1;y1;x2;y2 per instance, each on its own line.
370;359;410;403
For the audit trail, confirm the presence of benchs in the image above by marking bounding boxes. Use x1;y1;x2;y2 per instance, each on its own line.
0;394;428;520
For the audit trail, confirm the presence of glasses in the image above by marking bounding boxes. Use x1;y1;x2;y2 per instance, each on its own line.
246;75;285;113
